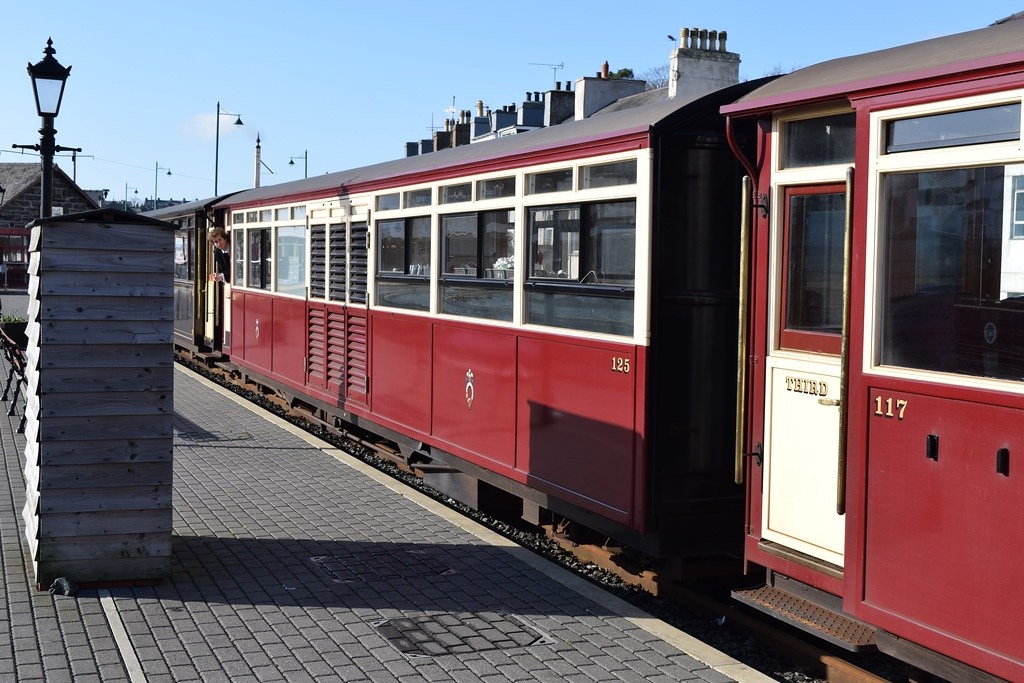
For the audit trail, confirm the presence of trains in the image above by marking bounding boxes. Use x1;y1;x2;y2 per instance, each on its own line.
143;12;1024;682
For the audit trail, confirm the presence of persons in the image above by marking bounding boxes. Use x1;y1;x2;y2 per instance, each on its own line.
207;227;231;282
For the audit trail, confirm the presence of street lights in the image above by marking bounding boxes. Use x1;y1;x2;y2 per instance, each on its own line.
289;150;308;178
213;101;245;197
11;35;81;215
153;160;172;208
125;182;139;210
